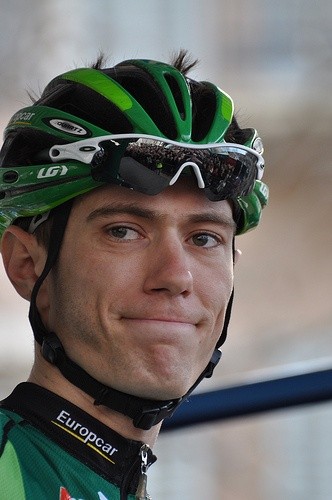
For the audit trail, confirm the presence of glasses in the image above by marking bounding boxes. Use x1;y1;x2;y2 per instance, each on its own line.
48;132;265;202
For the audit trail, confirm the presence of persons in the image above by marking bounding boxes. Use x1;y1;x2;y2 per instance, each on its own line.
0;47;273;500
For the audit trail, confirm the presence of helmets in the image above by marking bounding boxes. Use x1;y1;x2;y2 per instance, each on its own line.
0;59;269;246
